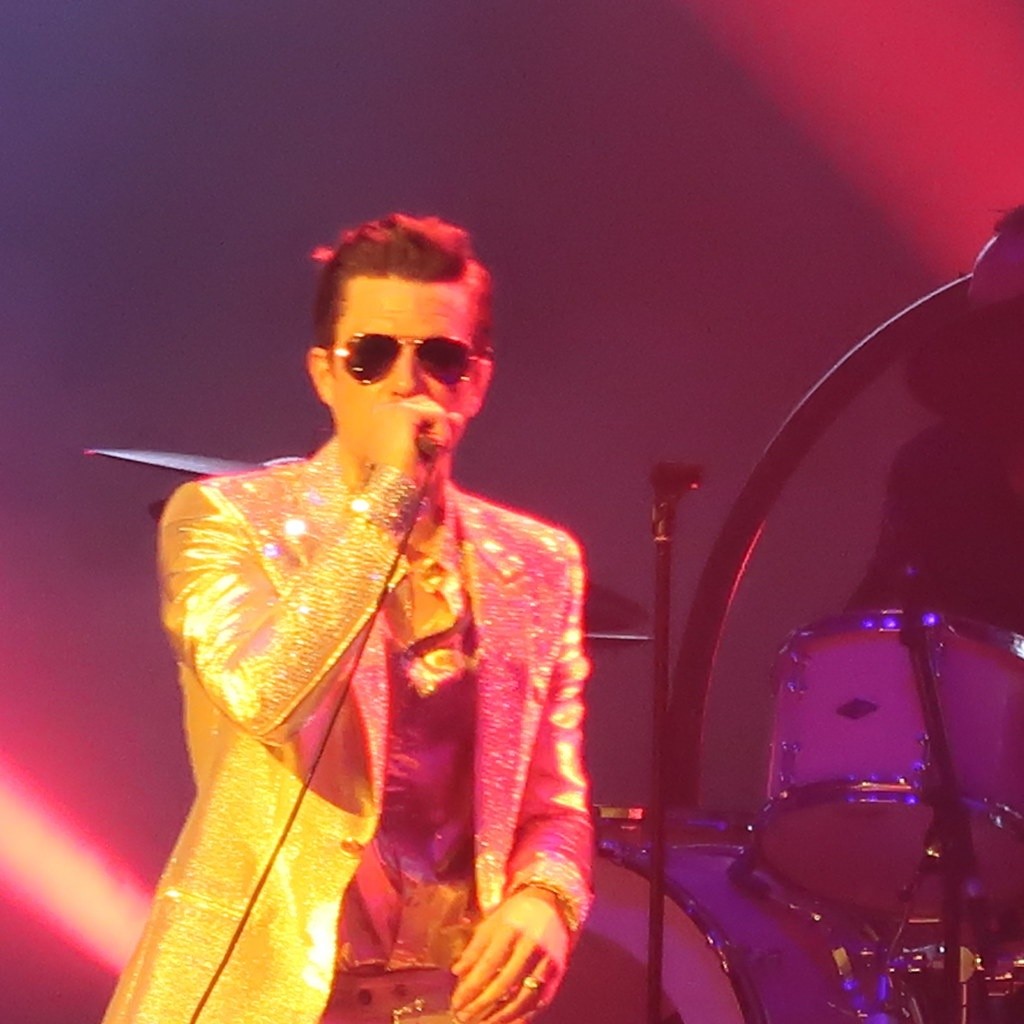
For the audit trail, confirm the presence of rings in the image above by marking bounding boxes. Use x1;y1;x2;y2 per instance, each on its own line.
523;976;542;991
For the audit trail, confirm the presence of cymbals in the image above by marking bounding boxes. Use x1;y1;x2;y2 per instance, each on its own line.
83;444;267;479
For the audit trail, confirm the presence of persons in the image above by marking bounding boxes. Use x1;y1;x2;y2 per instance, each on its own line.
843;202;1023;638
92;208;594;1024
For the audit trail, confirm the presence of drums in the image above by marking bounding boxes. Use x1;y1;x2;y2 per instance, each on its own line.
529;807;924;1023
751;606;1024;918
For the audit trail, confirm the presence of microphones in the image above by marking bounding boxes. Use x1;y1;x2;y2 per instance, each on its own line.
416;424;445;462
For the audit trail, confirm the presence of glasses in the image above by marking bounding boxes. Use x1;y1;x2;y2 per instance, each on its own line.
329;332;477;386
